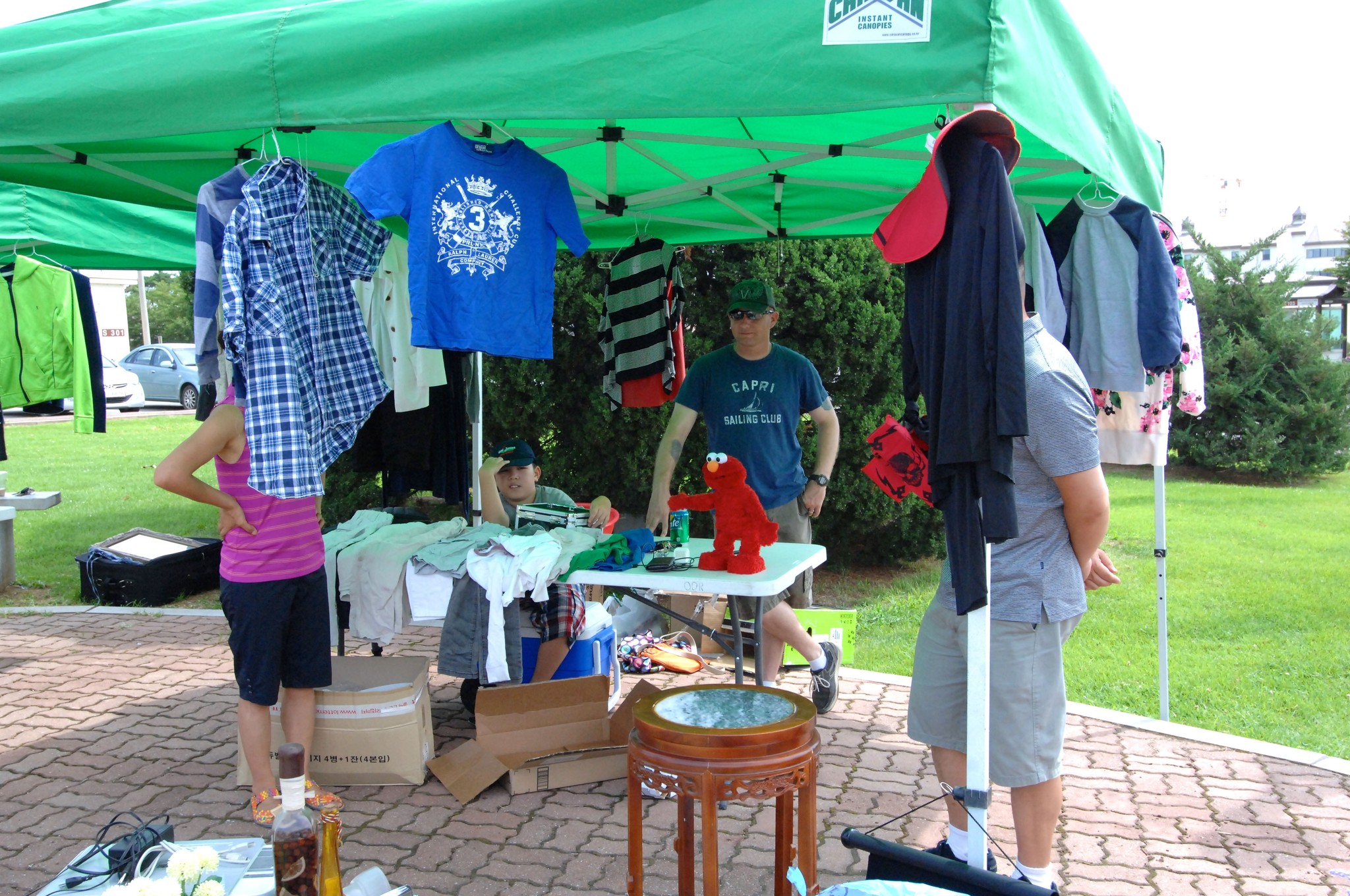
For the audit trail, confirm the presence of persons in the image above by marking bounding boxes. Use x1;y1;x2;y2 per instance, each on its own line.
646;281;843;714
480;444;612;683
155;330;345;827
904;305;1120;895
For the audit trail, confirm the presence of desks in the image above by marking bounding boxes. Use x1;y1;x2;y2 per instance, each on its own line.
324;524;833;810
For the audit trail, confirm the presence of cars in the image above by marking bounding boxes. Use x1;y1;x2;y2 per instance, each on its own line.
117;342;202;411
61;352;146;413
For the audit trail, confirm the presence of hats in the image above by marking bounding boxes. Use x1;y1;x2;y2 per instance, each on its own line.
489;439;535;468
727;278;776;313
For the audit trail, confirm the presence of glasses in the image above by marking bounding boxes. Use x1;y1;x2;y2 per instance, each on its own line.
729;310;774;319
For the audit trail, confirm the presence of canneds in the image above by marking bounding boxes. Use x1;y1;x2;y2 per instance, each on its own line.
669;508;690;544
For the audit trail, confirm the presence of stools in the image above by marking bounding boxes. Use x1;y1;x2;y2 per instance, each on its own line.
618;683;822;895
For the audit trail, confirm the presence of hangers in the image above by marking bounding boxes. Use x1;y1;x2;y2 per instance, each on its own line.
451;117;517;146
595;211;688;270
237;127;296;193
1080;174;1120;205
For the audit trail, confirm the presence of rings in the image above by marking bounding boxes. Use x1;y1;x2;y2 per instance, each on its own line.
808;511;814;514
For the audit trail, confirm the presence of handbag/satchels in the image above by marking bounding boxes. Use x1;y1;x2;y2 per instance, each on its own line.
640;638;710;674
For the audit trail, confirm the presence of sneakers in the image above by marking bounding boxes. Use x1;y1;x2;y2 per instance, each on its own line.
923;840;998;872
810;641;843;713
1003;873;1059;896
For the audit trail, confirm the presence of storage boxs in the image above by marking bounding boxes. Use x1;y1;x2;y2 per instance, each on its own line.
235;652;435;788
422;675;666;804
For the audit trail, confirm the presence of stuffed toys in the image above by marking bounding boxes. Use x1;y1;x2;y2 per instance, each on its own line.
667;452;778;574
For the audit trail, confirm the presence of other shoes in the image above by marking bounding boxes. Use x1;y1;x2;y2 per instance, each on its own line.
251;788;282;828
304;778;346;812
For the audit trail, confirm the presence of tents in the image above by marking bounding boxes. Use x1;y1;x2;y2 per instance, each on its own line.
0;181;198;348
0;1;1176;870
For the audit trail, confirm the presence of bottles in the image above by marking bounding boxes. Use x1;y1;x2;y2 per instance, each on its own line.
273;742;342;896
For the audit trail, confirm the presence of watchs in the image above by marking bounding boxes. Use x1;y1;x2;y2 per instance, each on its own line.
809;474;829;487
